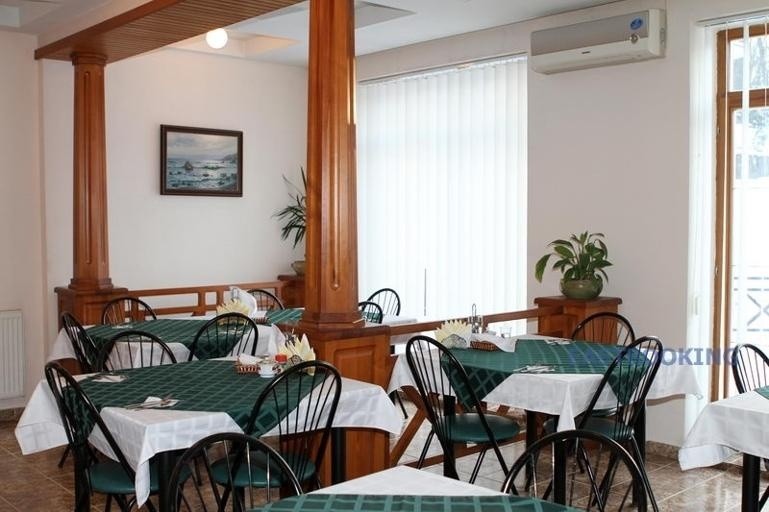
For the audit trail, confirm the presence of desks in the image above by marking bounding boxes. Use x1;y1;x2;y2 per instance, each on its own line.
264;309;379;328
711;387;769;512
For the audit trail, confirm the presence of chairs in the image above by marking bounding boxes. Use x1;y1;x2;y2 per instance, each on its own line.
524;311;636;494
45;364;193;511
361;287;401;316
405;335;520;497
102;297;157;326
210;359;342;510
100;328;177;373
500;429;648;512
538;336;663;512
732;341;769;396
246;288;286;311
358;302;384;326
169;432;303;509
50;310;109;468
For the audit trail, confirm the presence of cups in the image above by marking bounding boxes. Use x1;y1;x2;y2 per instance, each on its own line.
258;359;276;379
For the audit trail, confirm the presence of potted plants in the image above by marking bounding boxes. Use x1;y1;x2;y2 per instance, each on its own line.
271;167;309;276
534;231;613;300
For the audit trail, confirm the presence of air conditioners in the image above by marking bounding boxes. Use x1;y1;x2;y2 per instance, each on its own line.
530;10;666;73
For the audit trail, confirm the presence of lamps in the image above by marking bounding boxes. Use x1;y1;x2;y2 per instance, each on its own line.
207;28;230;50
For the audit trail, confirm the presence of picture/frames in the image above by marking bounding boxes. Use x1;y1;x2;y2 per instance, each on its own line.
160;125;243;198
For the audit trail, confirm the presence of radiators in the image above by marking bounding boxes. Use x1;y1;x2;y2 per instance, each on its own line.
0;307;26;397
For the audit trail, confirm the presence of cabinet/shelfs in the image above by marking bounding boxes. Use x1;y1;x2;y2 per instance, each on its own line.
535;297;623;451
